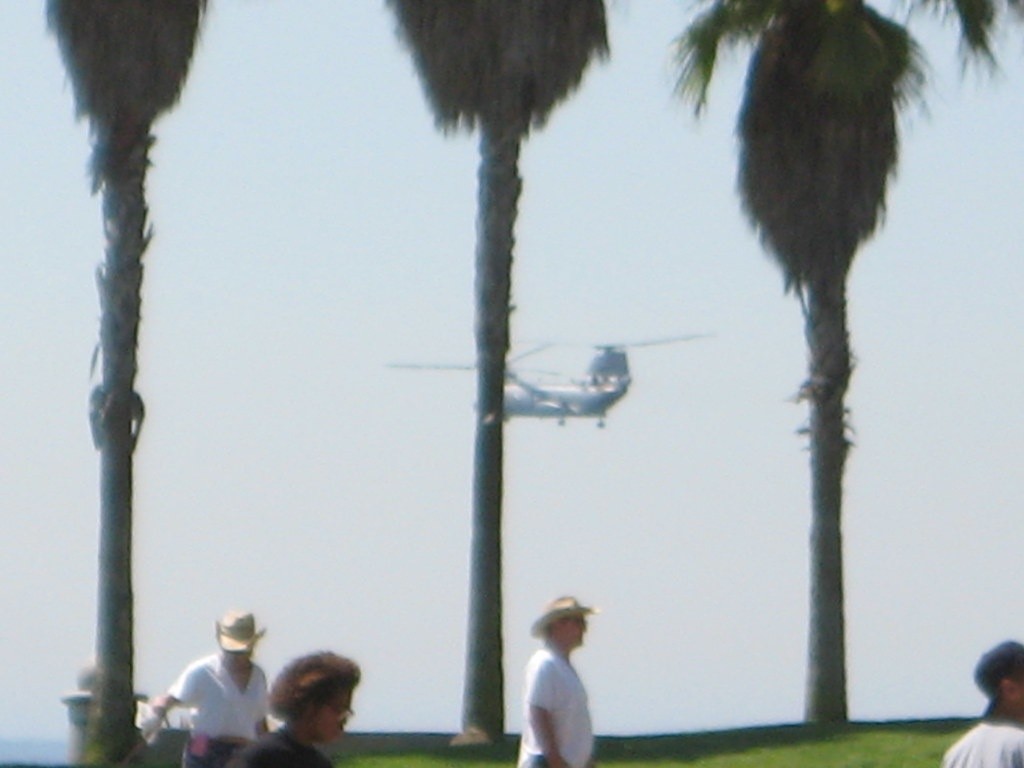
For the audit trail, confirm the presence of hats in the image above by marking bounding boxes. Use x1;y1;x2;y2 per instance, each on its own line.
60;662;148;702
215;608;268;652
528;596;598;639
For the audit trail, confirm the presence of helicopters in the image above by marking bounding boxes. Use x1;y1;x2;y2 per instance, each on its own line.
378;332;705;432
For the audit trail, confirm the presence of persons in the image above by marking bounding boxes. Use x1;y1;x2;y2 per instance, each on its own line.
516;595;595;768
941;641;1024;768
148;610;271;768
229;652;361;768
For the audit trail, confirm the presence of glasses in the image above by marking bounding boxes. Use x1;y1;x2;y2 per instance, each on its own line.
316;693;354;725
